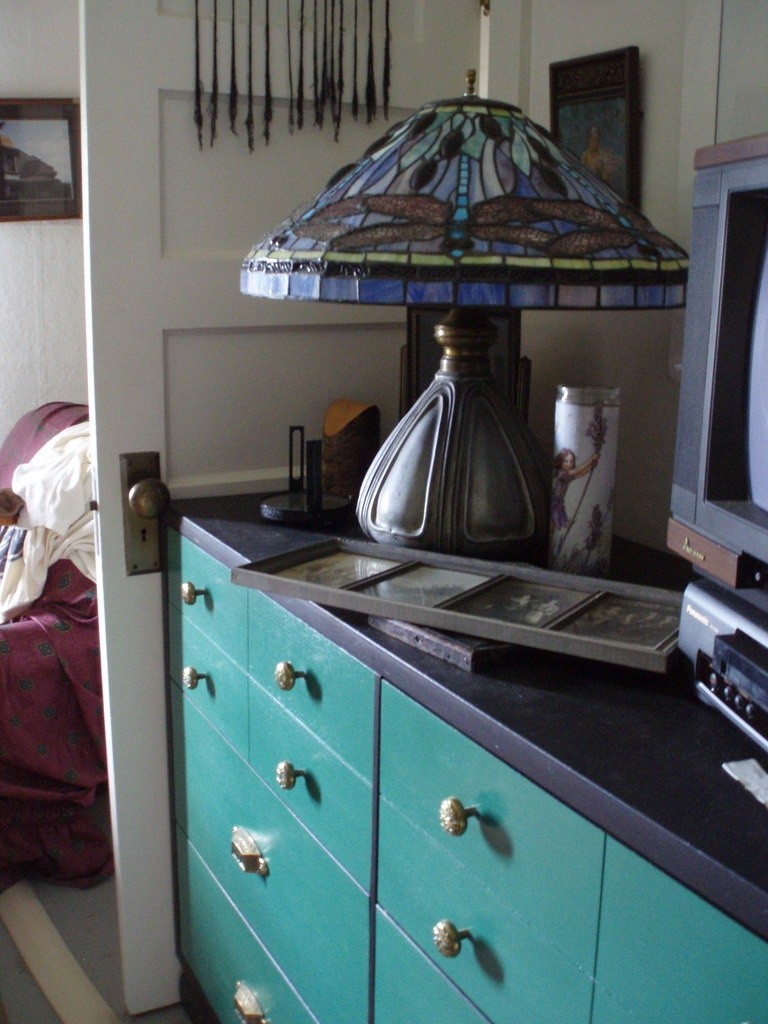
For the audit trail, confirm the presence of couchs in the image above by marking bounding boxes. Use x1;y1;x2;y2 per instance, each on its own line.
0;397;113;891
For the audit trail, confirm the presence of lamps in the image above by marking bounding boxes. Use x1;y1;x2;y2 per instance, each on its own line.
234;68;689;561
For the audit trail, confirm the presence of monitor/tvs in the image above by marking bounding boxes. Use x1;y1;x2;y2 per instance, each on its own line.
666;131;768;618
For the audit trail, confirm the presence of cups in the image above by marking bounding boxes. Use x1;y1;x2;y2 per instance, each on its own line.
550;380;622;582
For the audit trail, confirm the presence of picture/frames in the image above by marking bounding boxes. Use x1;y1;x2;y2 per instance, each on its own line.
0;99;80;223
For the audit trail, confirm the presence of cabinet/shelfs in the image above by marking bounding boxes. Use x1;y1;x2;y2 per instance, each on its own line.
162;527;767;1024
